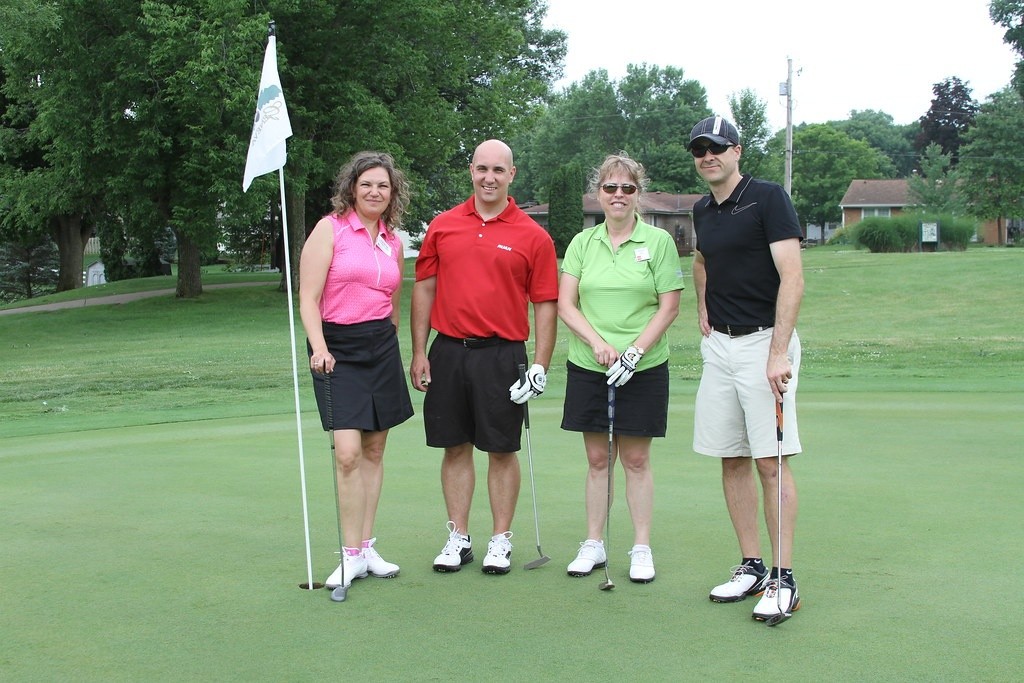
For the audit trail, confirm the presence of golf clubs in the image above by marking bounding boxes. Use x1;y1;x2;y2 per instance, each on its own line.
324;361;353;600
596;359;615;593
519;362;550;571
765;383;792;627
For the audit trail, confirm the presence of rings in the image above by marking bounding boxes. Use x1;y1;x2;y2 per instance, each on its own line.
783;378;788;382
315;363;318;365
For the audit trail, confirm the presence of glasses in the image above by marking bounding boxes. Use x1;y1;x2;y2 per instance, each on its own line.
691;143;737;160
600;182;640;195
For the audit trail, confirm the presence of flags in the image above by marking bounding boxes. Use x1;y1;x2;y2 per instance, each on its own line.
242;34;293;193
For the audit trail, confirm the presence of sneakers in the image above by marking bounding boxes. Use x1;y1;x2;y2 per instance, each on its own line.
709;561;770;602
433;520;473;572
362;537;400;579
752;571;800;619
482;530;513;574
325;543;368;591
628;544;655;582
566;538;607;576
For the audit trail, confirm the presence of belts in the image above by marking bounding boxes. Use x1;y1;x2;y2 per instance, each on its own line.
711;321;768;341
452;336;504;348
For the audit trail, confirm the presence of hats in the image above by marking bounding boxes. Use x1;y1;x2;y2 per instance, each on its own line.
685;116;739;153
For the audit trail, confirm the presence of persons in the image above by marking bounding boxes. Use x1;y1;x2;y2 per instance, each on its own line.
410;139;560;576
557;156;685;583
685;116;804;620
299;151;414;588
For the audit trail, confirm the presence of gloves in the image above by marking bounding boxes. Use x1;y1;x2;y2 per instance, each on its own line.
509;364;548;405
605;342;644;388
